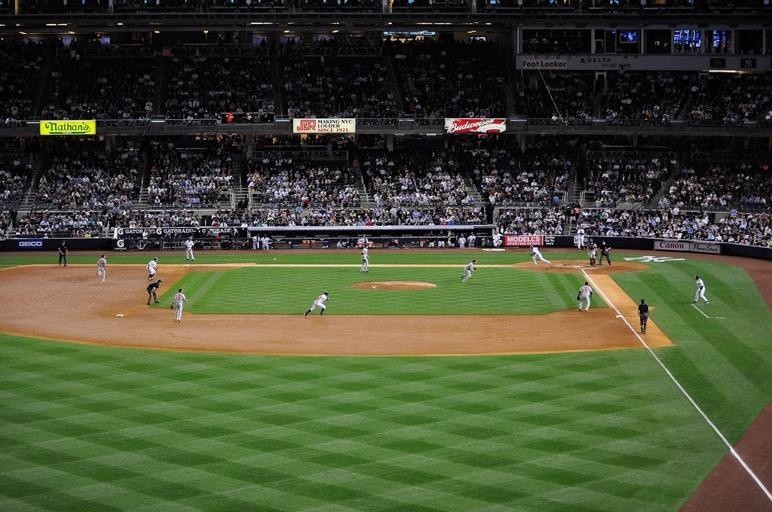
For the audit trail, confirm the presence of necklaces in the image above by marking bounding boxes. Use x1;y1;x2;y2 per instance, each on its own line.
360;245;369;272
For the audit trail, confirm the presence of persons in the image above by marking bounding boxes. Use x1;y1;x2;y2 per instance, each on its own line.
96;255;107;281
460;259;477;282
184;237;197;261
586;237;597;268
146;279;162;305
577;281;593;312
2;0;772;251
303;291;328;316
530;245;551;265
637;299;648;334
693;275;709;304
172;288;189;323
58;240;69;268
597;240;612;265
146;256;159;281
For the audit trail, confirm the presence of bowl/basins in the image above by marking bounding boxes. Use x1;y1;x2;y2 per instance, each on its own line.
360;245;369;272
